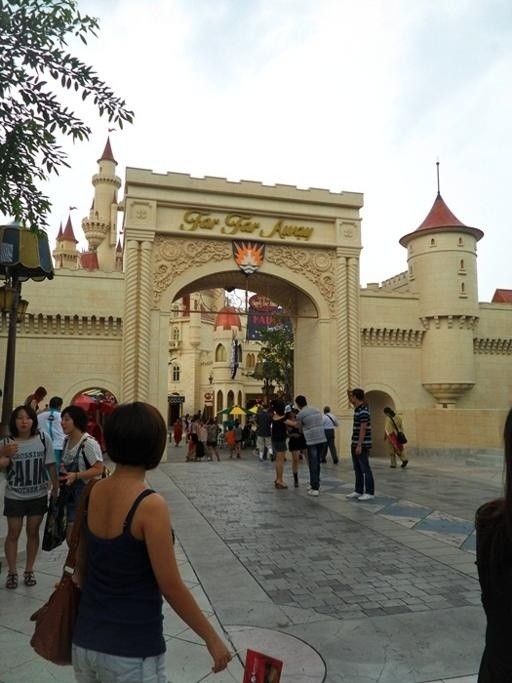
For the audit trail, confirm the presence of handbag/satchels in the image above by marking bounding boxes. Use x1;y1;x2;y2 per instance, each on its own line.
42;484;69;552
27;572;88;668
397;431;408;445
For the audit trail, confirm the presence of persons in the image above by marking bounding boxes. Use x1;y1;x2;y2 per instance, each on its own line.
0;404;60;589
23;385;48;415
383;406;409;469
470;407;511;682
37;396;69;490
70;400;234;682
171;386;376;501
55;405;105;547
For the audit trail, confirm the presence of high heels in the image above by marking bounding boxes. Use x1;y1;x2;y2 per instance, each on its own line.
274;479;288;489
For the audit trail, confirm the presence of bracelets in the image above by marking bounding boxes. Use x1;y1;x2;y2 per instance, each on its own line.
75;470;80;479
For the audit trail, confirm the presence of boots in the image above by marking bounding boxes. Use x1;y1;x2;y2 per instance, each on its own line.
293;471;299;488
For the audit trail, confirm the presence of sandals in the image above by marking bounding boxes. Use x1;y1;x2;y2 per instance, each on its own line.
5;572;19;590
23;569;37;587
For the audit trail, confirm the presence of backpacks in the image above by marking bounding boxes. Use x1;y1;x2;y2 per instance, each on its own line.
61;433;113;483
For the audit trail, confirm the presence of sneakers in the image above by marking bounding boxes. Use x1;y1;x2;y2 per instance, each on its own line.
307;485;320;497
357;493;375;501
344;491;362;499
390;459;408;468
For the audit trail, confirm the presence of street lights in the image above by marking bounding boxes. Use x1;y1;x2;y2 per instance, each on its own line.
0;281;31;439
262;378;276;405
